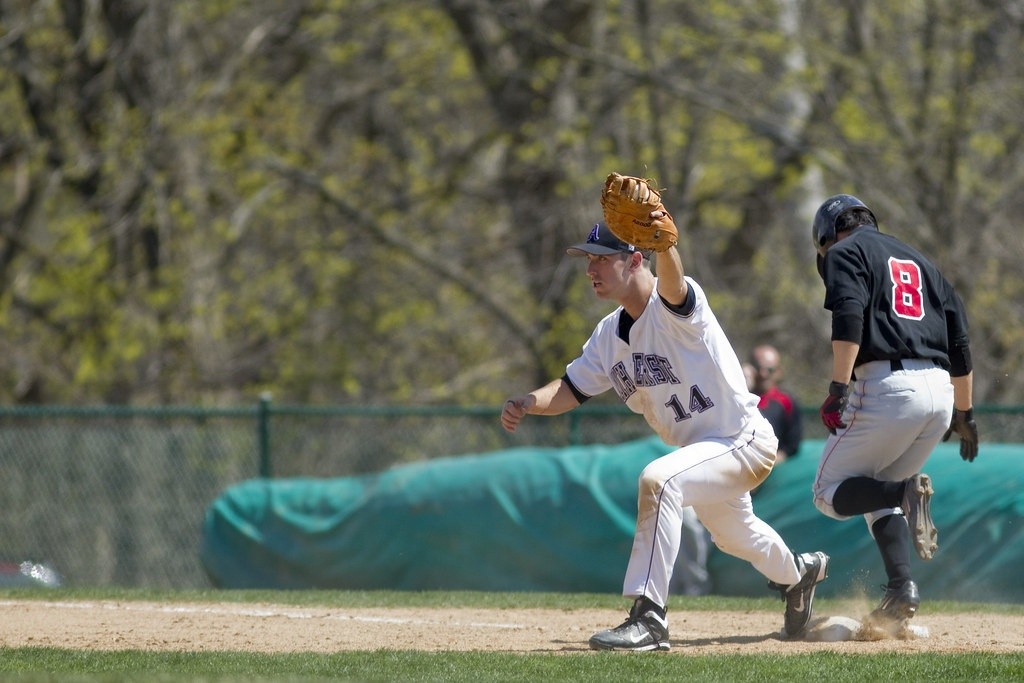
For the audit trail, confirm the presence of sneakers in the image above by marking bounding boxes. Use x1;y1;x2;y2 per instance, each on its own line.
861;579;920;635
767;551;831;639
588;596;671;651
899;472;939;560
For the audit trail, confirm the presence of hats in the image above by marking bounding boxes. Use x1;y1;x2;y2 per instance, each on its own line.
565;219;651;261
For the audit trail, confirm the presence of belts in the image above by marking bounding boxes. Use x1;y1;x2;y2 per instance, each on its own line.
850;358;942;382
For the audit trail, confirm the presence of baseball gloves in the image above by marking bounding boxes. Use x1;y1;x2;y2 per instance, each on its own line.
601;171;680;252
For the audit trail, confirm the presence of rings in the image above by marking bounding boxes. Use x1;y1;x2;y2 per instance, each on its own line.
507;400;516;406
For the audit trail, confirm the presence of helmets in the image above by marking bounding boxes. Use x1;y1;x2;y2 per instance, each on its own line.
812;193;880;260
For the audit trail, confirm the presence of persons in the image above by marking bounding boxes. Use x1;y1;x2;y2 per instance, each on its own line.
749;346;802;465
500;172;829;651
811;195;979;622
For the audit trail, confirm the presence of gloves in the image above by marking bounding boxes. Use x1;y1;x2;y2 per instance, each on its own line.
818;380;850;436
942;404;979;463
